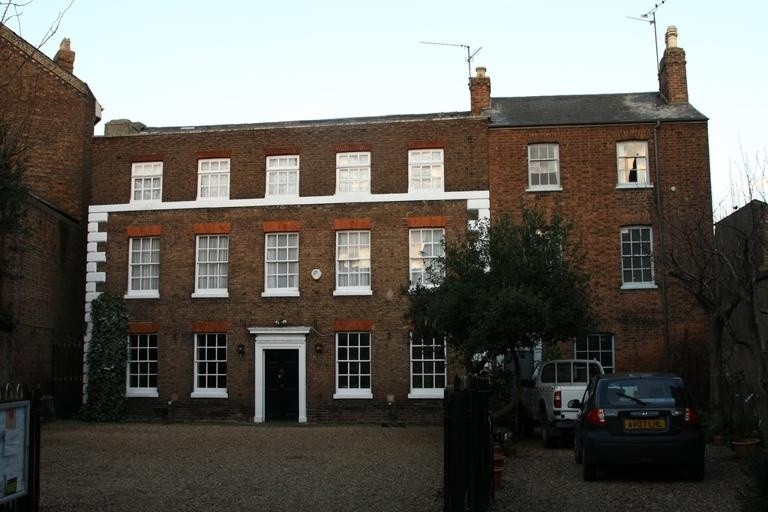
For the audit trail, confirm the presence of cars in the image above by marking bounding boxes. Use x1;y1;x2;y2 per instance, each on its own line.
572;372;706;481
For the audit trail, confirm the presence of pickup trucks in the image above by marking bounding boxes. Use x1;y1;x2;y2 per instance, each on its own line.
517;359;640;445
